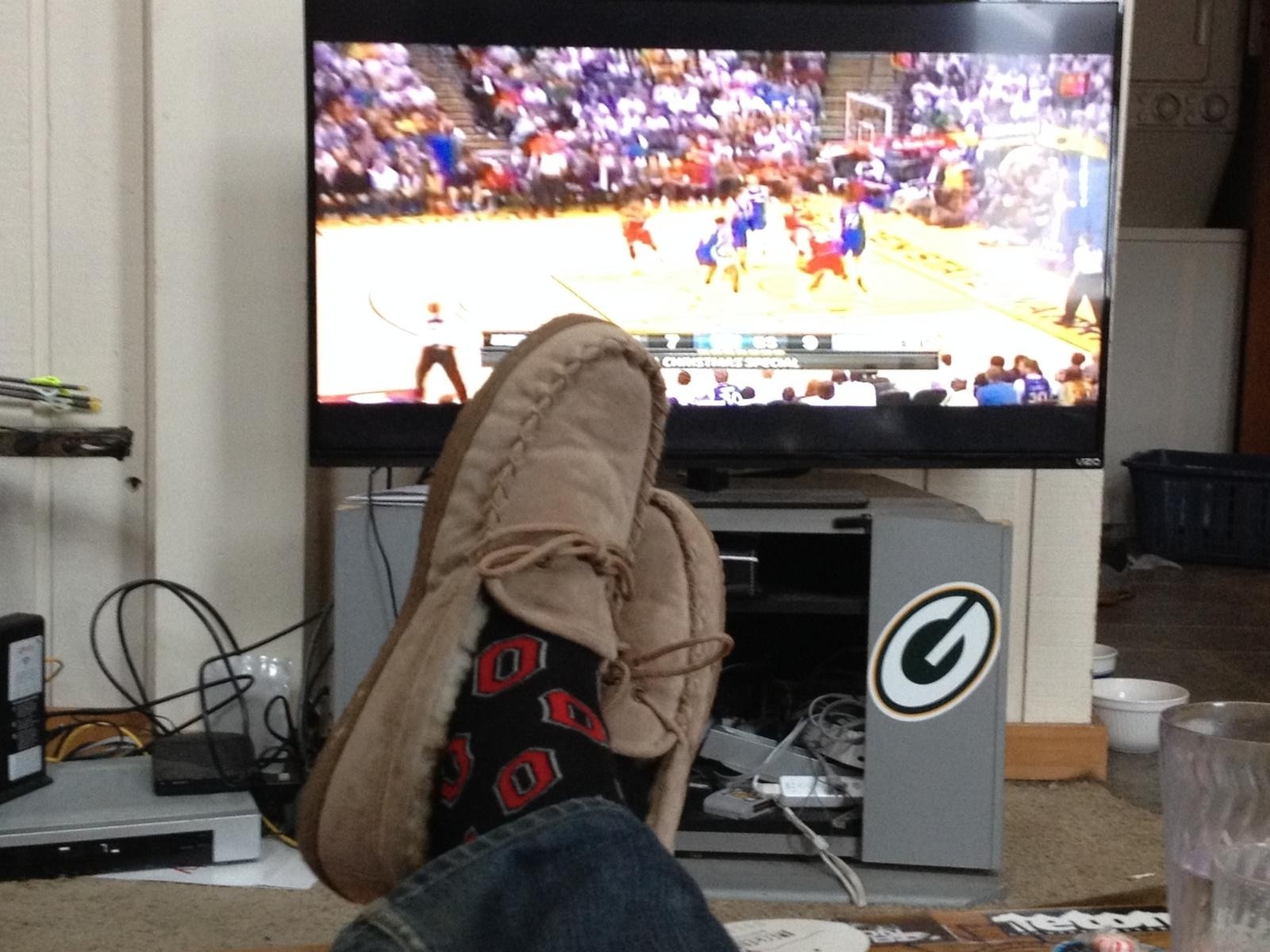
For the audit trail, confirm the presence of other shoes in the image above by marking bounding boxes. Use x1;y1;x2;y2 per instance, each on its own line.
601;487;731;860
294;315;669;914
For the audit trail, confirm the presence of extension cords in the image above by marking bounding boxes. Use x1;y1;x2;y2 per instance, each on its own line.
775;774;864;807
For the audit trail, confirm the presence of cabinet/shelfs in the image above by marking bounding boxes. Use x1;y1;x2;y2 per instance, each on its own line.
331;472;1014;907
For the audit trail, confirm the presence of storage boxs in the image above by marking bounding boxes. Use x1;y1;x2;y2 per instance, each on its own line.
1121;447;1270;572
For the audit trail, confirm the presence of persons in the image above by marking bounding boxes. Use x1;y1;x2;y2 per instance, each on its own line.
288;312;751;952
311;38;1117;412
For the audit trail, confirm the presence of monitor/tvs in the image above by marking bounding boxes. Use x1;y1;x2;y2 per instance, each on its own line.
302;2;1129;468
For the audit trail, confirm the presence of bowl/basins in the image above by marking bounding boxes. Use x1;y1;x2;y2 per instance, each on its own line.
1092;677;1191;754
1092;643;1118;679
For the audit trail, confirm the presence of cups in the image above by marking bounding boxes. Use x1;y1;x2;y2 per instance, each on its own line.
1212;841;1270;952
1158;701;1270;952
